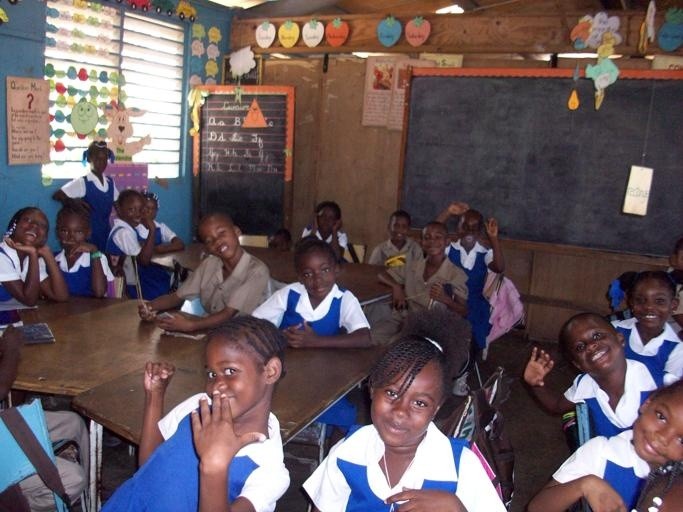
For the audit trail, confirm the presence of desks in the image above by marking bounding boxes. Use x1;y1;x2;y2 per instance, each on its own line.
74;331;391;511
0;298;220;410
155;244;399;310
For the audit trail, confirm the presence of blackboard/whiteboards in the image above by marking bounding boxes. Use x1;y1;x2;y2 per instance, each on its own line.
191;84;294;250
396;68;680;269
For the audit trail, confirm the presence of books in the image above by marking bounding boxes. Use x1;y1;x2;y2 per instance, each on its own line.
24;322;54;346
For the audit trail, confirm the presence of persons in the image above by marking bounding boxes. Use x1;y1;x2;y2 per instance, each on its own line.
434;202;504;360
0;206;70;331
609;269;683;387
522;314;682;443
665;241;683;315
526;378;683;511
99;318;289;512
0;322;91;512
303;201;348;265
52;141;118;262
302;337;506;512
51;204;115;299
253;241;374;348
368;211;423;268
362;223;467;345
138;212;273;333
135;192;184;297
107;189;172;299
